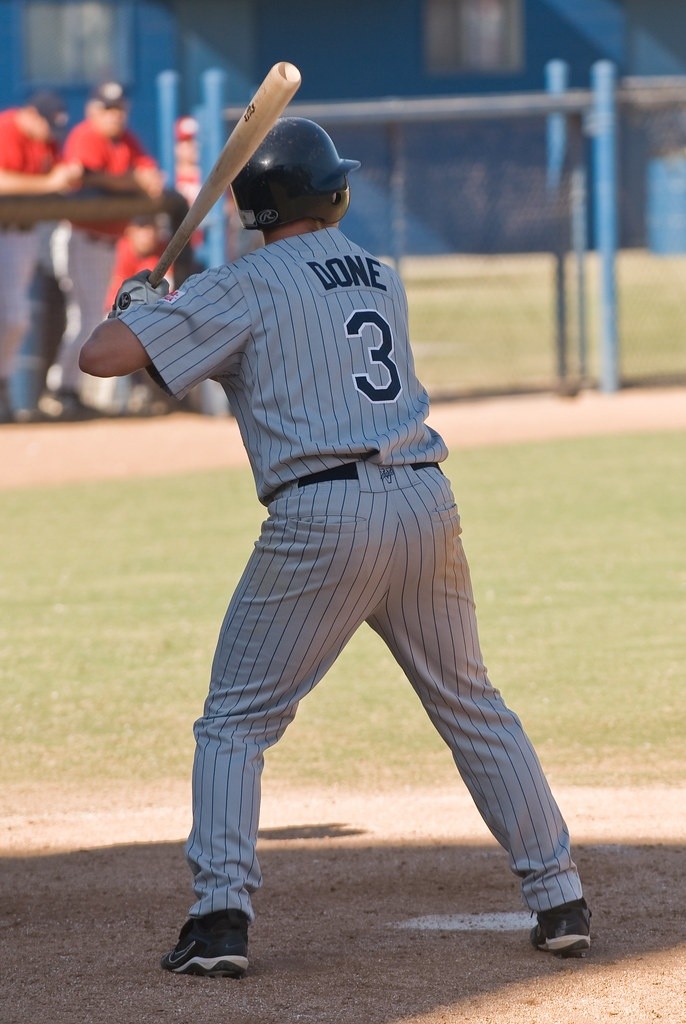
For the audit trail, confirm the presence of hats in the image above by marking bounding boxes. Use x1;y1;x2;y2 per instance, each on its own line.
30;90;69;132
90;81;127;109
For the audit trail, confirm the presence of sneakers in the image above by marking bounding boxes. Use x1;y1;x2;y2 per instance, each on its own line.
160;909;250;979
530;896;592;958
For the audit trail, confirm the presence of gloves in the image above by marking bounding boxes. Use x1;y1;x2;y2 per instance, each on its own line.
107;269;170;320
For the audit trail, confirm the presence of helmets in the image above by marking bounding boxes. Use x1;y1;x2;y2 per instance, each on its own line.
229;116;362;229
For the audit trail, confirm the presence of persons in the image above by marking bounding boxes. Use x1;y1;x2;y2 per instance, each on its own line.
0;84;242;420
79;119;591;979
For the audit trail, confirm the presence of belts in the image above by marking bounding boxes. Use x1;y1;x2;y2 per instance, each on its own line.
298;462;440;488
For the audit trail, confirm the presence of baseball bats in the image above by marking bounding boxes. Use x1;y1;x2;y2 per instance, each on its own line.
147;62;302;289
